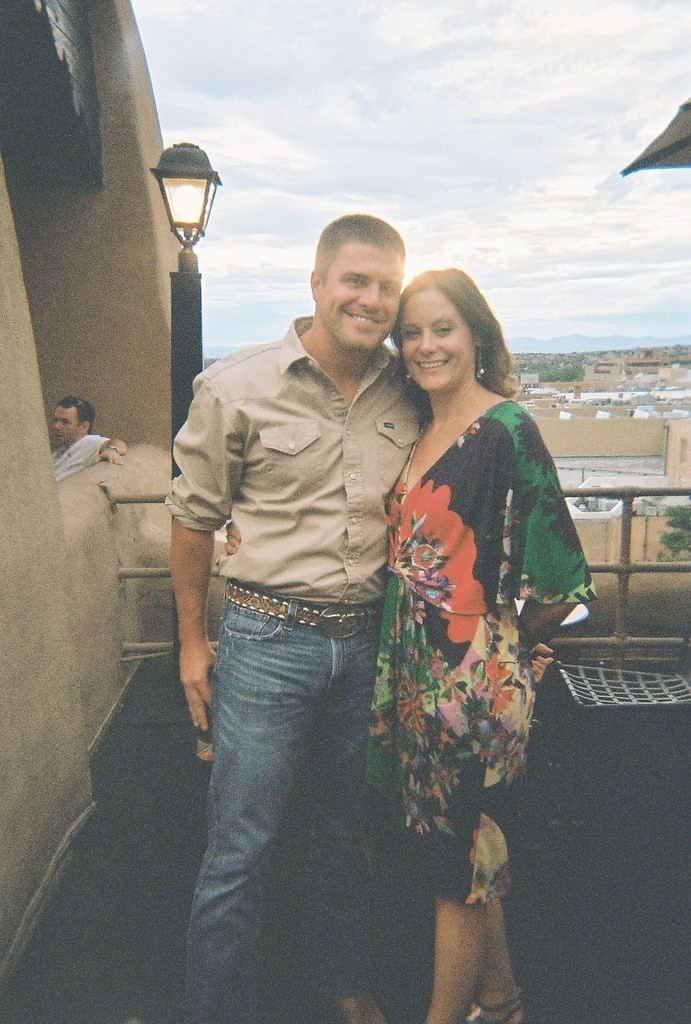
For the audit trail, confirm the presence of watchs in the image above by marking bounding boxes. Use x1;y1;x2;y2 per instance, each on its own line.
106;444;118;452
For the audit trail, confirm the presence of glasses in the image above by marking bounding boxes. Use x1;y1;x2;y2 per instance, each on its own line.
65;396;85;411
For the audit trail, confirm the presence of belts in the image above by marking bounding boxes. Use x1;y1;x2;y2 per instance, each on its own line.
226;584;381;639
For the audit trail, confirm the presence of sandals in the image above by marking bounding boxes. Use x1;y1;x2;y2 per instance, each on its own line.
462;990;527;1024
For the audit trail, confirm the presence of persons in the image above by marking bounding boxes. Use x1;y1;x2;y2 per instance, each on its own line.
224;269;598;1024
52;396;128;482
165;214;555;1024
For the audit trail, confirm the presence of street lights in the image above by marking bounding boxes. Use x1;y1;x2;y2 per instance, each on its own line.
149;143;222;685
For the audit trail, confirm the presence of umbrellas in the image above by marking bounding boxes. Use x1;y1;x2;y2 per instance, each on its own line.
620;98;691;177
